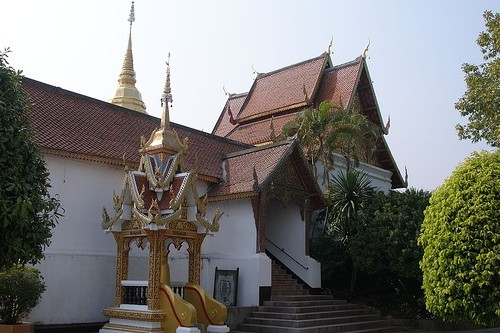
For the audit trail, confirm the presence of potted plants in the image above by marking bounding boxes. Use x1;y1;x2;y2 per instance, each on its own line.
1;265;47;333
